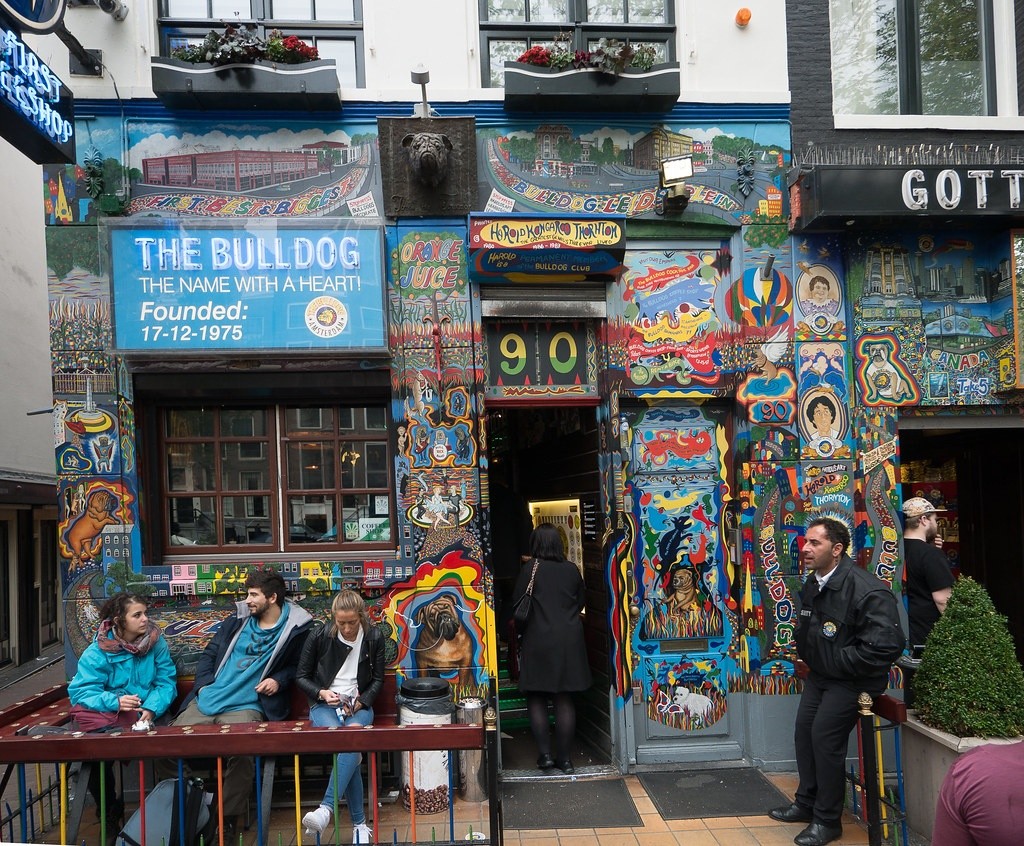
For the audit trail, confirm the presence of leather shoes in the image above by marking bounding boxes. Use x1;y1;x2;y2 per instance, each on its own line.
536;752;555;768
552;758;574;774
794;821;843;846
768;803;815;823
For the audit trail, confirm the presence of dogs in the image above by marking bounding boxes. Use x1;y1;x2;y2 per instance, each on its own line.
402;132;454;186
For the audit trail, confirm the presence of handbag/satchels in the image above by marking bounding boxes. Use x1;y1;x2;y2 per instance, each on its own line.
512;558;539;635
505;619;527;683
70;698;140;733
115;776;218;846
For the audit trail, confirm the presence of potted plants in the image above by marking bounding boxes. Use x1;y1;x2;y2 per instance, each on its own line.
901;573;1024;840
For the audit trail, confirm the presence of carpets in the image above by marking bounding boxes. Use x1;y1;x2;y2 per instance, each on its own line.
636;768;792;821
500;778;645;830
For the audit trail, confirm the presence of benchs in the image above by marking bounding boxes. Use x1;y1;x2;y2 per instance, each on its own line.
161;674;399;809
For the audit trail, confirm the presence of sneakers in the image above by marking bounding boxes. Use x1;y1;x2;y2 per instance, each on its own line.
301;804;334;839
352;823;373;845
211;814;236;846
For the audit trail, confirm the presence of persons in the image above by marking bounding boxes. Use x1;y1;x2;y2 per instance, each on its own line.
156;571;315;846
511;523;594;774
768;518;906;846
931;738;1024;846
67;592;178;837
294;587;385;842
902;497;957;709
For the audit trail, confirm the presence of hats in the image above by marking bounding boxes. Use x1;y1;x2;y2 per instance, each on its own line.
901;496;949;518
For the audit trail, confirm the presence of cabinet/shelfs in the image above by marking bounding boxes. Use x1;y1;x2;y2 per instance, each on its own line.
500;645;557;731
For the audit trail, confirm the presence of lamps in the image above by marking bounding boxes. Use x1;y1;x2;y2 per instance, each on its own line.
411;69;435;119
658;153;694;212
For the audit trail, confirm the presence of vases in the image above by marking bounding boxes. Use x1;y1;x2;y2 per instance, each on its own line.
151;56;343;112
504;61;680;113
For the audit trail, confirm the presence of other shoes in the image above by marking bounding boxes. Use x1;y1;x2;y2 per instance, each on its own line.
97;798;123;846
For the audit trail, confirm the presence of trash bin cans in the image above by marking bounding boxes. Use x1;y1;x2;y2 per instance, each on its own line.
455;696;489;803
896;644;925;709
399;676;457;813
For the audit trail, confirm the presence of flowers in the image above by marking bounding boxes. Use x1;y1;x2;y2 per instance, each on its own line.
170;12;321;64
517;31;657;76
